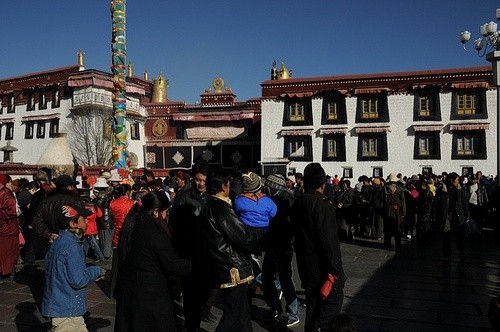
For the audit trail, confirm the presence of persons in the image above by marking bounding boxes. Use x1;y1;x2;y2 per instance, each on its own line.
260;172;305;205
329;313;359;332
228;175;238;203
168;170;219;322
113;191;182;332
326;170;500;255
262;174;302;328
36;203;112;332
0;167;193;279
233;172;278;242
290;163;347;332
199;170;263;332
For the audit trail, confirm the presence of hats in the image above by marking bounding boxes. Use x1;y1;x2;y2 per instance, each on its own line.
57;203;93;225
371;178;380;185
54;175;80;188
108;174;123;182
285;175;295;184
342;178;351;188
302;163;326;191
92;178;109;188
470;174;479;180
0;173;8;184
388;173;400;182
99;171;111;179
264;174;287;191
240;171;262;194
409;175;420;181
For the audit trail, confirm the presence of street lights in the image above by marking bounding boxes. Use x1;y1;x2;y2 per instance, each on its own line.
458;8;499;183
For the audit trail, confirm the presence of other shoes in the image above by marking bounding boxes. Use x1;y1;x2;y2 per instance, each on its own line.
277;288;283;301
279;315;301;328
261;309;284;321
